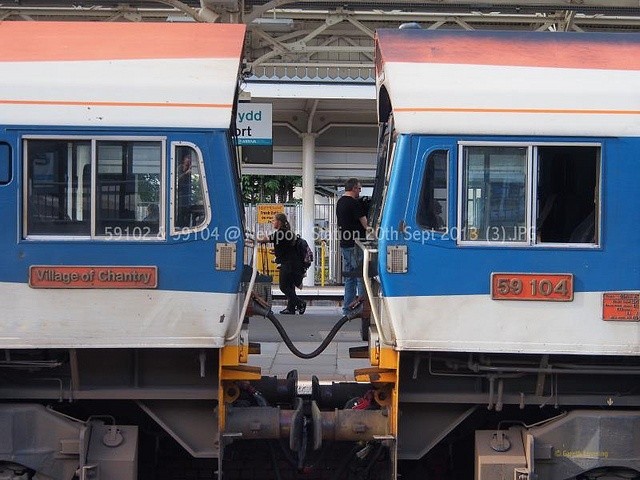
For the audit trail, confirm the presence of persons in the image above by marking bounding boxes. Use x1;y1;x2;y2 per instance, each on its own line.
434;202;444;226
336;178;372;318
144;204;159;220
244;213;307;315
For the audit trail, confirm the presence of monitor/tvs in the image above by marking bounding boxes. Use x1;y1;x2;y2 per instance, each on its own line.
241;144;274;165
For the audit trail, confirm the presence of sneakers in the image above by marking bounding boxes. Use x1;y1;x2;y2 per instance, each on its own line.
279;308;295;315
298;301;307;313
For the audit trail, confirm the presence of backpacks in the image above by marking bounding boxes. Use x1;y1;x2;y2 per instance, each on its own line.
295;236;313;269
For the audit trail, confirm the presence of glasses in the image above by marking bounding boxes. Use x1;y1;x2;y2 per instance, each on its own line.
354;186;362;189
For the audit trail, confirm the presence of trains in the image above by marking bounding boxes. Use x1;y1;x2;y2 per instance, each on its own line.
0;21;640;479
